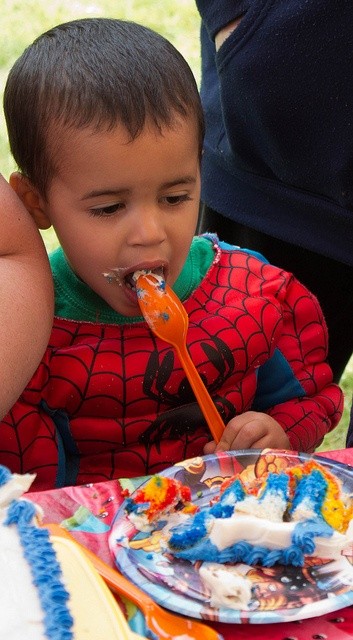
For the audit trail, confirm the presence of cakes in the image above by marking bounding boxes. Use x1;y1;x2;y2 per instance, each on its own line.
169;470;335;569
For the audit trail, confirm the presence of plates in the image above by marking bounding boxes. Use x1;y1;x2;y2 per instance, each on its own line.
108;449;353;624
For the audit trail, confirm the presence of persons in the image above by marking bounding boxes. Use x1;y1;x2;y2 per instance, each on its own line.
0;172;55;422
0;18;344;493
194;1;353;385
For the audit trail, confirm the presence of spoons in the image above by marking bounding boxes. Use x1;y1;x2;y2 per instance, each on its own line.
135;273;227;445
